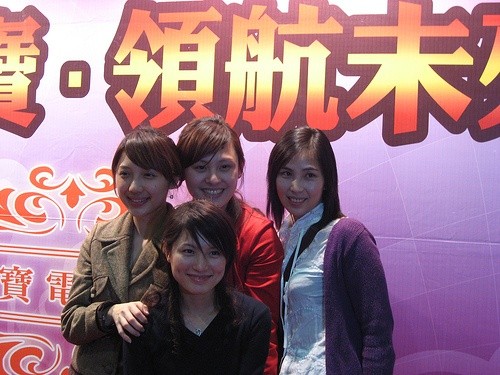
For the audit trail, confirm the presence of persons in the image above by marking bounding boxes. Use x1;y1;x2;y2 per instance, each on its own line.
121;200;270;374
176;120;285;375
61;130;184;375
266;127;395;375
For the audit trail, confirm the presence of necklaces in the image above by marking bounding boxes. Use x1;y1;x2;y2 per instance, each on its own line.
179;301;215;335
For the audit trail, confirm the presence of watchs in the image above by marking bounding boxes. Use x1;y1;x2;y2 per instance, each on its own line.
97;300;113;332
